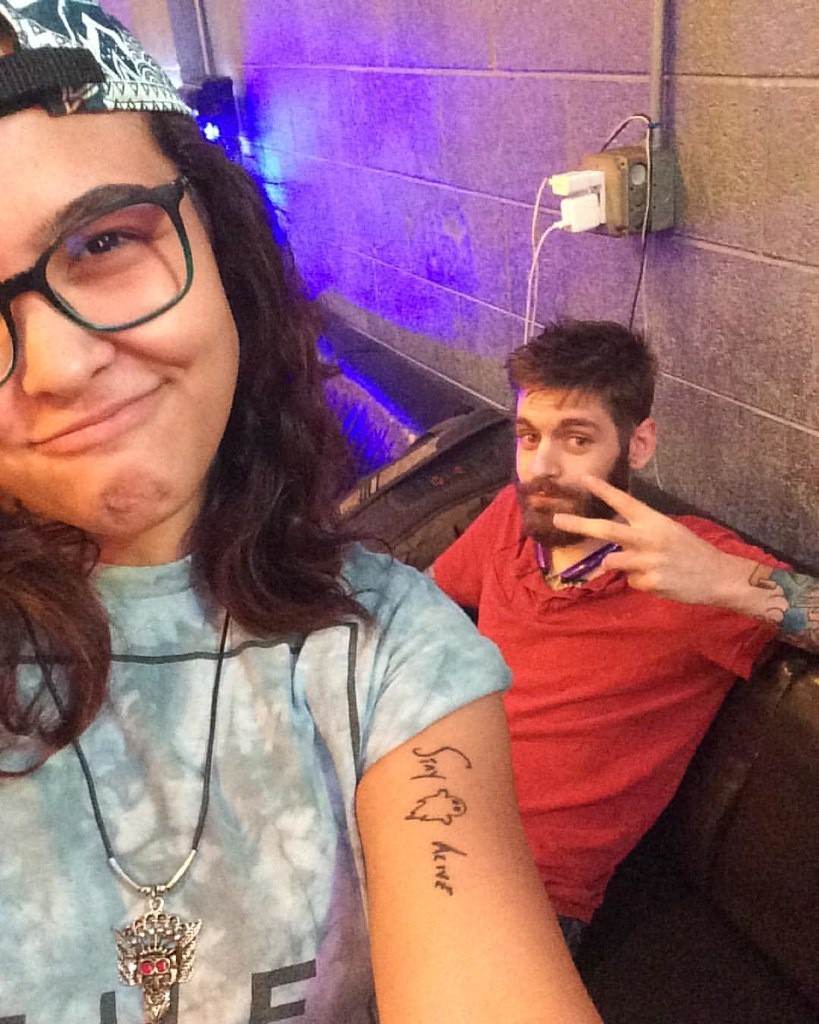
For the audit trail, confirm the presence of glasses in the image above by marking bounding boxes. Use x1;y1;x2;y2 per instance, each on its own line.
2;172;192;388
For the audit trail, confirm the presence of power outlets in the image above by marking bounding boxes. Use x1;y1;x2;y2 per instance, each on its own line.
577;144;677;238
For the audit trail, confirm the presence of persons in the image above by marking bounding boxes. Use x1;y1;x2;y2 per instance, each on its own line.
424;318;819;962
0;0;601;1023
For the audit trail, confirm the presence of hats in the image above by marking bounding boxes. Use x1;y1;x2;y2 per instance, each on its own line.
0;0;199;121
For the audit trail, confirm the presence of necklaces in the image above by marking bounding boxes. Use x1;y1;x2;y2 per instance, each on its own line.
2;584;232;1023
535;540;623;584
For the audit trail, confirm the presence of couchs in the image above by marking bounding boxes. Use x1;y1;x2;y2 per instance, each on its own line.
307;403;819;1024
